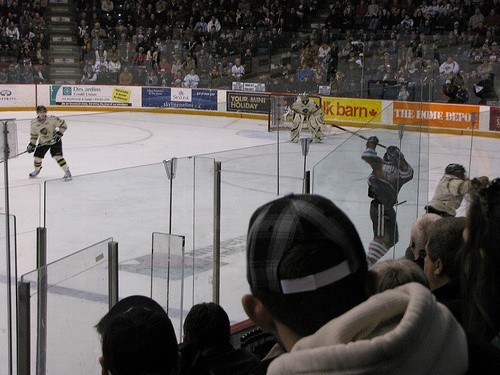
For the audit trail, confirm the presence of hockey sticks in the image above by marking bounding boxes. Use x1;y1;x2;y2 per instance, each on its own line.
330;122;388;150
0;137;55;164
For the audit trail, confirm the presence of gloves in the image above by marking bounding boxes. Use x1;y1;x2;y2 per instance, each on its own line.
26;143;35;153
367;136;379;145
53;131;64;142
480;175;488;186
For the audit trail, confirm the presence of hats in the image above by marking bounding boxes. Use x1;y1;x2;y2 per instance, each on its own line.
246;193;366;301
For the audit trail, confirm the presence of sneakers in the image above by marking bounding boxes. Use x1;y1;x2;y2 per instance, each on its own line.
62;170;72;182
29;171;40;178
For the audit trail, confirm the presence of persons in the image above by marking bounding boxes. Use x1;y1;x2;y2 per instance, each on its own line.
96;297;182;375
361;135;414;265
179;302;264;375
2;0;498;105
424;212;470;322
240;192;468;374
27;104;73;179
366;255;432;298
424;163;487;215
455;179;500;374
282;93;326;145
404;212;445;272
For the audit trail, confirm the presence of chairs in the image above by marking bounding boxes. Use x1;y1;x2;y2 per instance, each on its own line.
0;0;500;108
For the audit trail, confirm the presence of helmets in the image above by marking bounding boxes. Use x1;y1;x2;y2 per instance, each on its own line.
301;96;308;101
384;146;404;162
445;163;465;177
35;106;47;114
